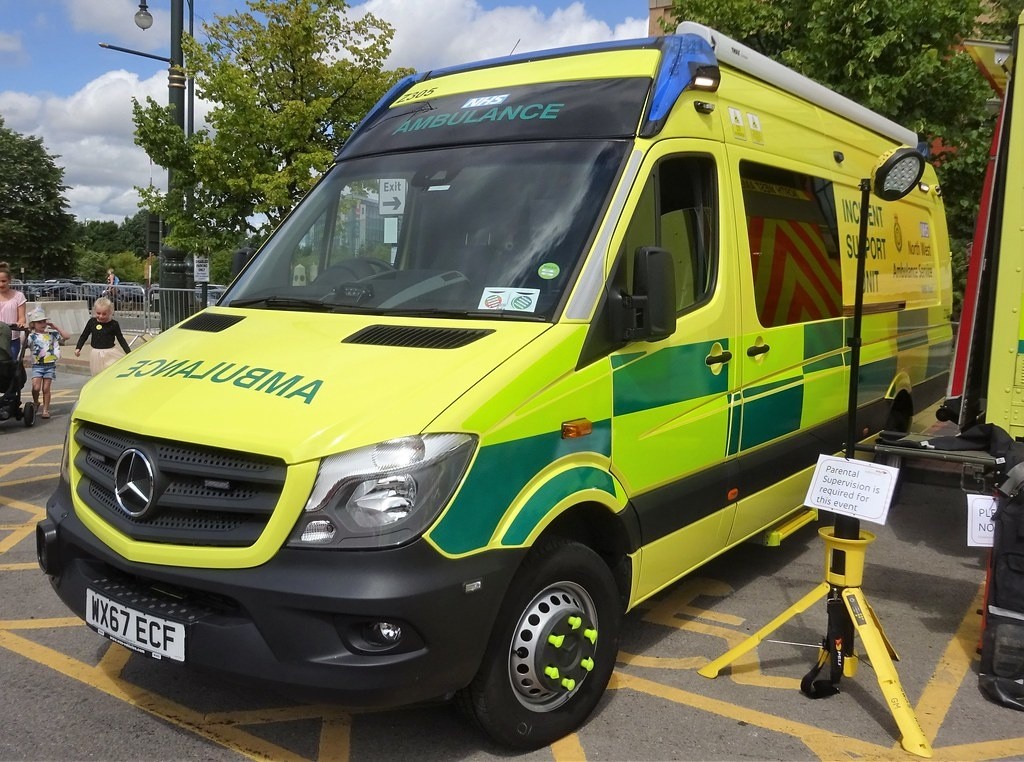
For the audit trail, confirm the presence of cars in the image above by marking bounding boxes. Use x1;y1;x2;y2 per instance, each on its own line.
195;283;227;291
194;288;208;307
208;289;226;308
9;278;160;310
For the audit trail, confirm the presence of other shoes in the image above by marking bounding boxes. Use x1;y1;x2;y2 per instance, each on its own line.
41;409;51;418
33;401;41;414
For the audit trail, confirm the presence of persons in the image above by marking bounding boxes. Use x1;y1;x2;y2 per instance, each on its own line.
104;269;122;311
0;262;27;360
73;297;132;378
21;306;71;418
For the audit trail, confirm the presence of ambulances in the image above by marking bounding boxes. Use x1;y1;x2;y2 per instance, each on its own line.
35;20;954;753
883;12;1024;712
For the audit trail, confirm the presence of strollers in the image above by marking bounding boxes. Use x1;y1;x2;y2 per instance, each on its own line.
0;321;35;427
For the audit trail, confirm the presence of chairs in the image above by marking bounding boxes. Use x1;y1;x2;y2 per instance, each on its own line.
426;179;508;279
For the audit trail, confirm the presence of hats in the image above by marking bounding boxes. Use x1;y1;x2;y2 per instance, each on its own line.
27;308;51;323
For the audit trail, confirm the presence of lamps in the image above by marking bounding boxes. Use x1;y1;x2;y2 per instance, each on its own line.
870;145;928;201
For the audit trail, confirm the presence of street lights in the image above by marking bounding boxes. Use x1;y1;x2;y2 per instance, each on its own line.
98;0;193;319
